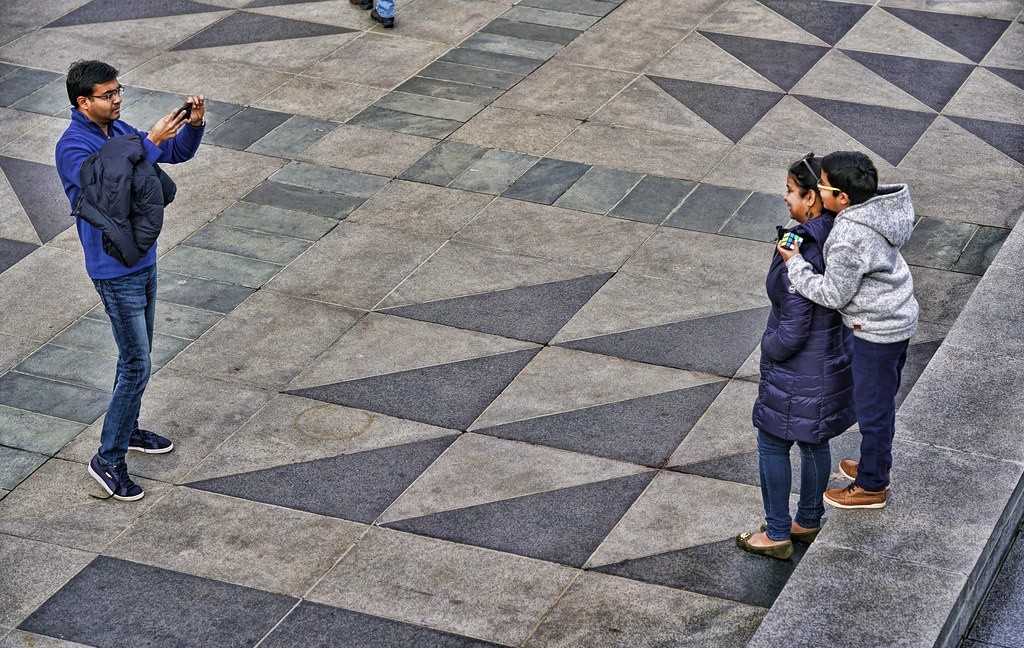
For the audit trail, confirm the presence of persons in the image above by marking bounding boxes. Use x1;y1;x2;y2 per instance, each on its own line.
735;152;920;558
350;0;395;28
53;59;205;505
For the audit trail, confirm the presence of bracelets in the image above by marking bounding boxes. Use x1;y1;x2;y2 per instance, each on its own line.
201;115;205;126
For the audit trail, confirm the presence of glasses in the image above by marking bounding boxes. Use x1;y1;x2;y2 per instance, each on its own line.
795;153;819;182
816;178;850;203
82;84;125;101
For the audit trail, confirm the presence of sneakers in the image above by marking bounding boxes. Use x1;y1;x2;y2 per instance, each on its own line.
126;428;174;454
823;481;887;509
88;454;145;502
839;457;890;491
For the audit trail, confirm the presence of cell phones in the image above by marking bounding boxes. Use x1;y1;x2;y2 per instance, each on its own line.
172;103;192;125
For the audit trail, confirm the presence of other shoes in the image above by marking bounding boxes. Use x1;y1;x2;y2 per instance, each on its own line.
371;8;395;28
736;532;794;560
760;522;822;543
349;0;374;10
